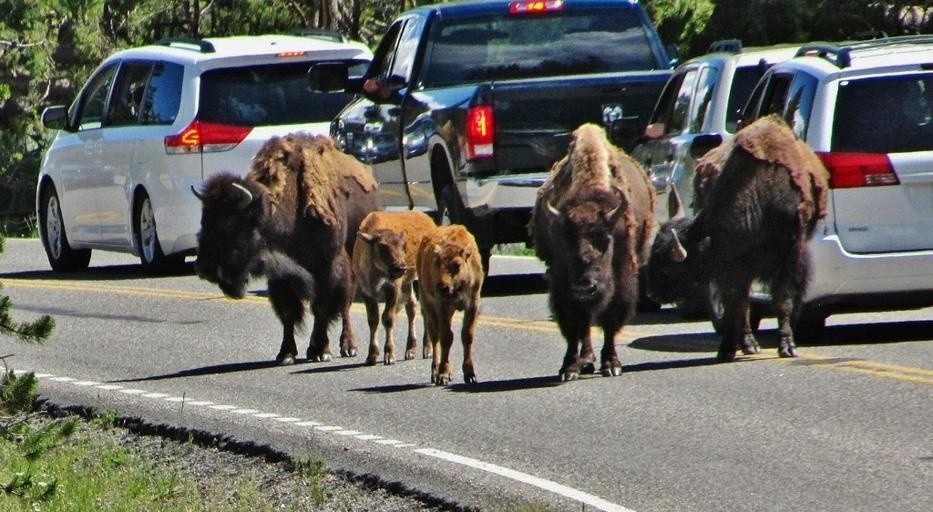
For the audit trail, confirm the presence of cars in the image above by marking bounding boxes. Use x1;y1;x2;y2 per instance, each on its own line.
35;28;376;275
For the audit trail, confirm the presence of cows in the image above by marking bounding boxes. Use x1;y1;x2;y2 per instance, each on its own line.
415;224;485;387
190;130;386;366
351;206;439;366
526;122;659;382
645;112;832;363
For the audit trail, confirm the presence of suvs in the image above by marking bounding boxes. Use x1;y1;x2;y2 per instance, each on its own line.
305;1;678;284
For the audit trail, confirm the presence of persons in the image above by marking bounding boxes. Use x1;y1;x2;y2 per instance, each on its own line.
363;79;381;92
646;123;665;137
